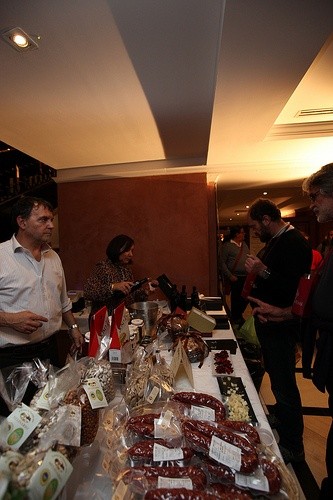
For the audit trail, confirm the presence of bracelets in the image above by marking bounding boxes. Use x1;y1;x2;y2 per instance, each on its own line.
111;283;114;291
260;268;271;279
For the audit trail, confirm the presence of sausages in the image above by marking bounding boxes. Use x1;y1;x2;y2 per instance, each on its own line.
123;391;281;500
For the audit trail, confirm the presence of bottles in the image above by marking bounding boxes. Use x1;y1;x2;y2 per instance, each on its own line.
115;277;148;300
128;319;146;354
170;284;199;314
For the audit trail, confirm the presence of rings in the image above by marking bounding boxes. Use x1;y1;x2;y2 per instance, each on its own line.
128;286;130;288
124;291;125;293
126;289;128;292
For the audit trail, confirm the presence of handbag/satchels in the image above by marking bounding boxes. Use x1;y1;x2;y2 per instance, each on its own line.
223;284;231;295
291;244;330;317
312;333;330;393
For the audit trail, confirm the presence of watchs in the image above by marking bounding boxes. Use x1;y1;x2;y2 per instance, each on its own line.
67;324;79;333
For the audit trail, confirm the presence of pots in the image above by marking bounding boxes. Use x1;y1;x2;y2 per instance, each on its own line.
128;302;160;339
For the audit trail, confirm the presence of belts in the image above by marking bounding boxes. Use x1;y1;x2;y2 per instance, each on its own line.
0;332;60;354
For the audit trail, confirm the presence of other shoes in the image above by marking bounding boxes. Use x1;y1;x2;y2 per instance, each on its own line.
303;372;313;379
277;441;305;465
267;415;278;429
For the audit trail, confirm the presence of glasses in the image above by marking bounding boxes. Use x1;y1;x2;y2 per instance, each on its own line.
309;191;320;201
240;232;244;234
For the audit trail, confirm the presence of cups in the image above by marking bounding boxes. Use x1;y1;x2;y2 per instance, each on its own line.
257;428;275;450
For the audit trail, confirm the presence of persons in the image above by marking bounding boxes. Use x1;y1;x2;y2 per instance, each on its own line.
221;225;250;326
250;198;312;466
0;196;89;406
307;228;333;259
83;235;159;331
247;162;333;500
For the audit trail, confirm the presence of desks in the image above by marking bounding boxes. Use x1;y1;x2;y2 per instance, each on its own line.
174;297;285;464
